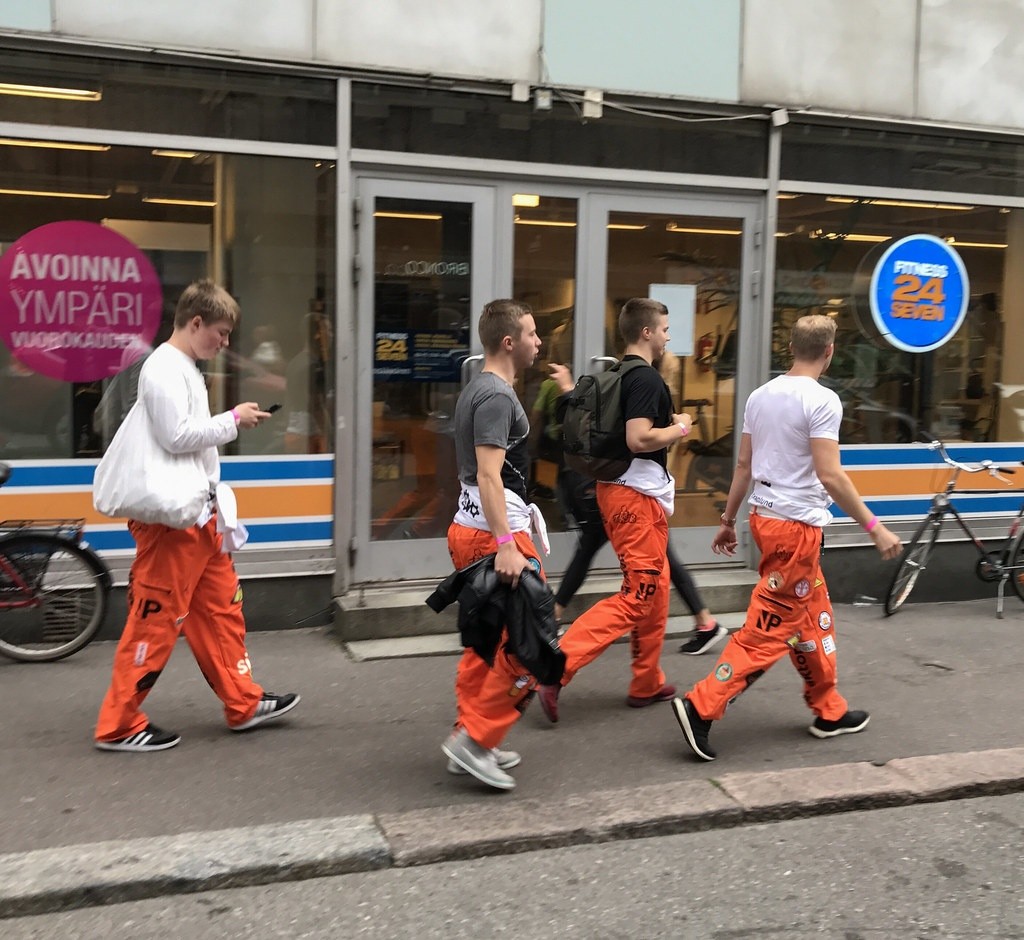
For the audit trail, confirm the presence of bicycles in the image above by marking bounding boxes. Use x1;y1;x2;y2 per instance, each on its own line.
0;463;114;663
884;431;1024;618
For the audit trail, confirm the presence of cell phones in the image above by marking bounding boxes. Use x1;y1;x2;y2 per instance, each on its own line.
265;404;283;412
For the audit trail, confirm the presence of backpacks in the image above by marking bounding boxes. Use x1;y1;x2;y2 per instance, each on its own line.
562;358;656;480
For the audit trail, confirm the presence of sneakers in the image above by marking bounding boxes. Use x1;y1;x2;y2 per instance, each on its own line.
445;743;521;774
626;684;677;708
672;696;717;762
439;724;516;790
229;692;301;730
94;722;180;752
555;617;565;636
807;709;871;738
680;618;728;654
537;680;562;724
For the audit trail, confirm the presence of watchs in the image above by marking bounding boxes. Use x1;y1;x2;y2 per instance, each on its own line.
721;513;736;526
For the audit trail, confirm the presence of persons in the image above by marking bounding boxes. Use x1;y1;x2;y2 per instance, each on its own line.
533;374;565;443
119;301;176;422
545;356;727;655
92;278;301;752
283;317;334;450
537;297;693;721
372;421;455;539
674;315;903;759
441;298;546;791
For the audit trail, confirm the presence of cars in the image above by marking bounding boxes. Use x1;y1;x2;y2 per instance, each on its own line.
768;372;918;443
0;324;288;433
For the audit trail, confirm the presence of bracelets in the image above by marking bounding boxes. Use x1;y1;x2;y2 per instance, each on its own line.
678;422;688;436
231;409;240;426
496;534;514;545
865;516;879;530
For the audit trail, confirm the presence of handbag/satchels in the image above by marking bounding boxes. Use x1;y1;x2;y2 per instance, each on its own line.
91;352;210;530
94;369;124;456
